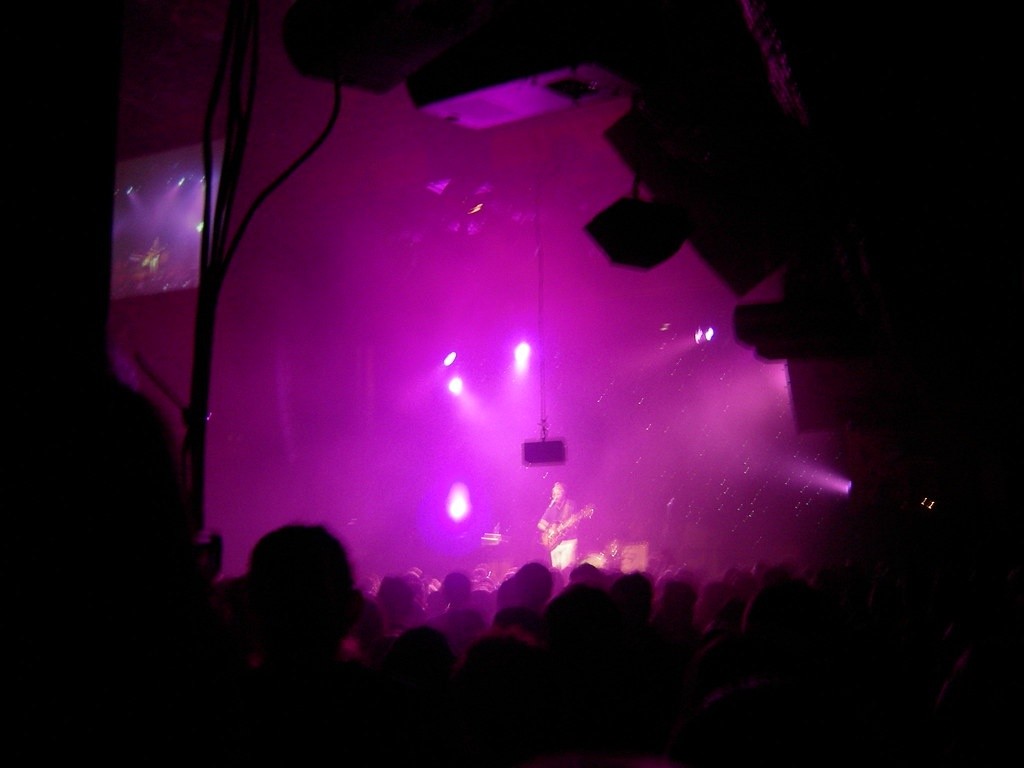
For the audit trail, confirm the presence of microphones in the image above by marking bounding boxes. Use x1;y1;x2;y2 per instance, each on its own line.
549;500;556;508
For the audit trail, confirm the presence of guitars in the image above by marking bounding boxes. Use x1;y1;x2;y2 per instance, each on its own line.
542;504;595;553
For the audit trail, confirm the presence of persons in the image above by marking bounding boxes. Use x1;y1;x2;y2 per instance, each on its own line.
537;483;580;568
196;503;1024;768
147;237;162;272
